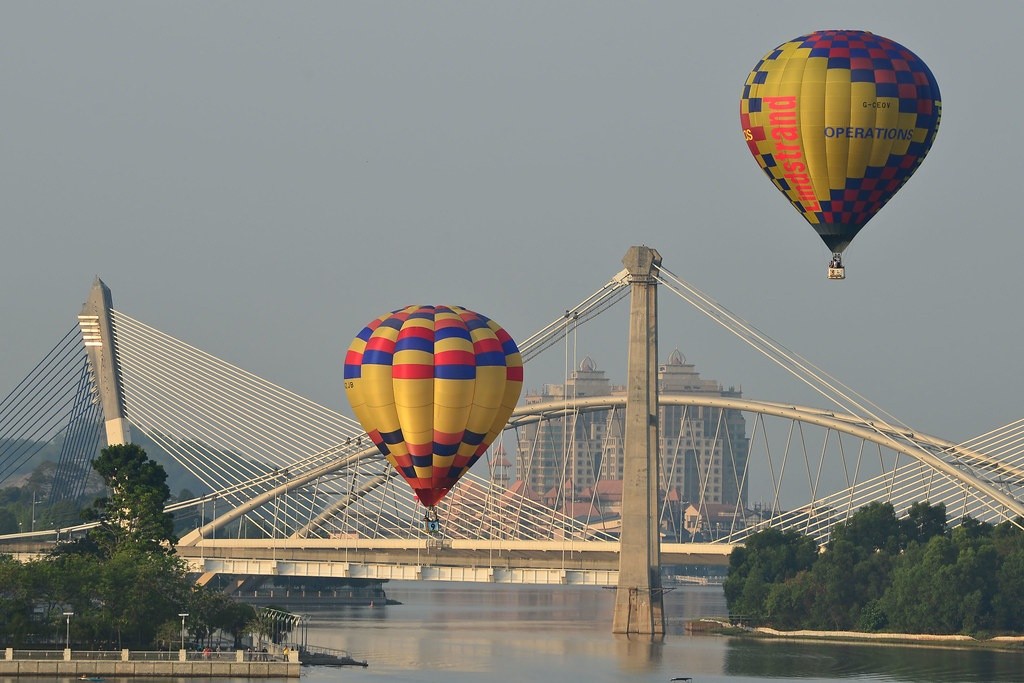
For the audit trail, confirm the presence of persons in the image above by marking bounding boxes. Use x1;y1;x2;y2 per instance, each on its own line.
830;259;838;268
202;644;290;662
426;509;437;521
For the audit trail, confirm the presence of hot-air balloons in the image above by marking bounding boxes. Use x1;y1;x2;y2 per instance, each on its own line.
341;304;524;536
739;29;941;281
